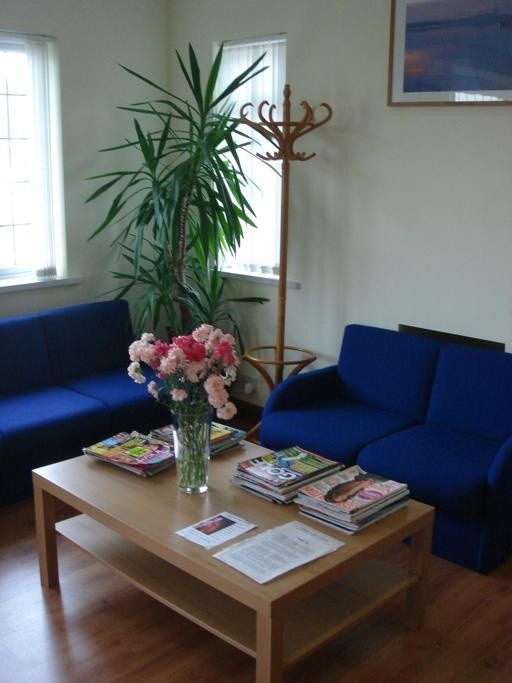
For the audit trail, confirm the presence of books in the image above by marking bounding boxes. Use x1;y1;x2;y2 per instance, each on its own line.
226;446;411;535
84;421;245;478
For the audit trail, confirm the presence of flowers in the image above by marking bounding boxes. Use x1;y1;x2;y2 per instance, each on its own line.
126;324;240;486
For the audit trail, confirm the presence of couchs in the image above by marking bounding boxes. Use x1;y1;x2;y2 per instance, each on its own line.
258;324;512;576
0;299;170;510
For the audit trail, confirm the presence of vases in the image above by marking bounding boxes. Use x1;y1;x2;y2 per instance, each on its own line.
169;409;217;494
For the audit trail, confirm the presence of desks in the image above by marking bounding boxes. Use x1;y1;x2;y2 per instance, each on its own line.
30;438;436;683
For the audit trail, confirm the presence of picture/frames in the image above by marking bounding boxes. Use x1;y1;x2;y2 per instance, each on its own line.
386;0;512;107
